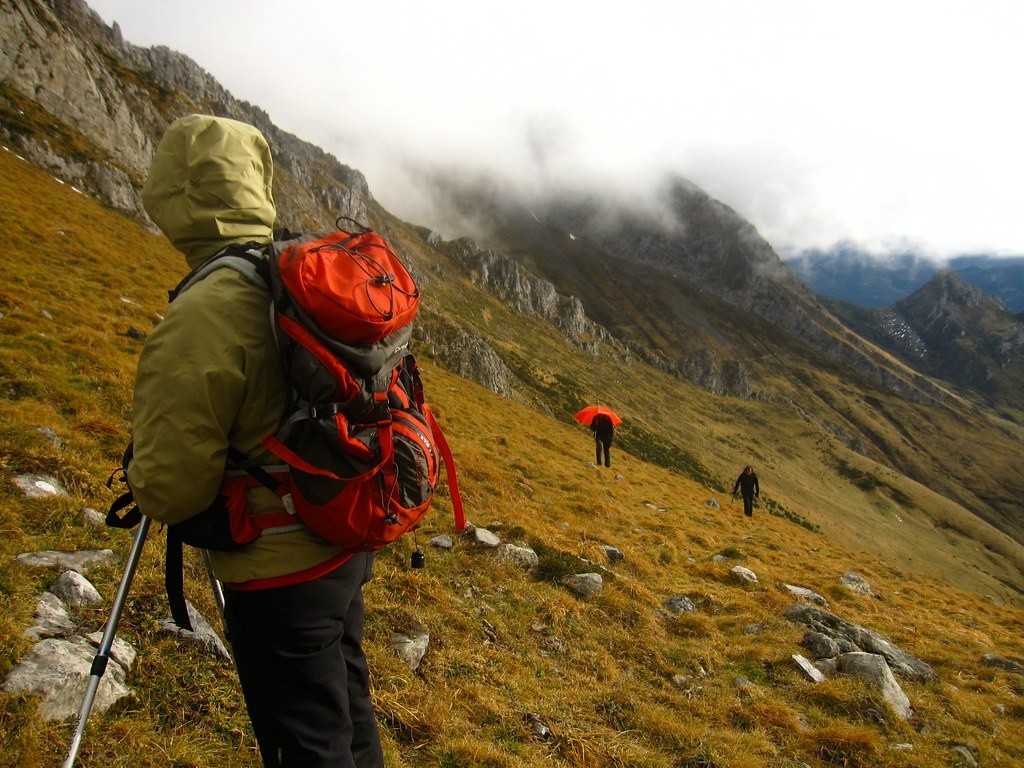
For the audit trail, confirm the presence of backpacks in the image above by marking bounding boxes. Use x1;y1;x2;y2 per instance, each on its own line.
105;214;468;631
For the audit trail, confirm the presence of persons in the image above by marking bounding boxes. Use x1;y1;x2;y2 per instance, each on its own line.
126;115;384;768
590;414;614;468
733;466;759;517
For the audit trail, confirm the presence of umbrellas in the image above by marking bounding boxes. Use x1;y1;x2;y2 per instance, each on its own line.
573;406;622;425
